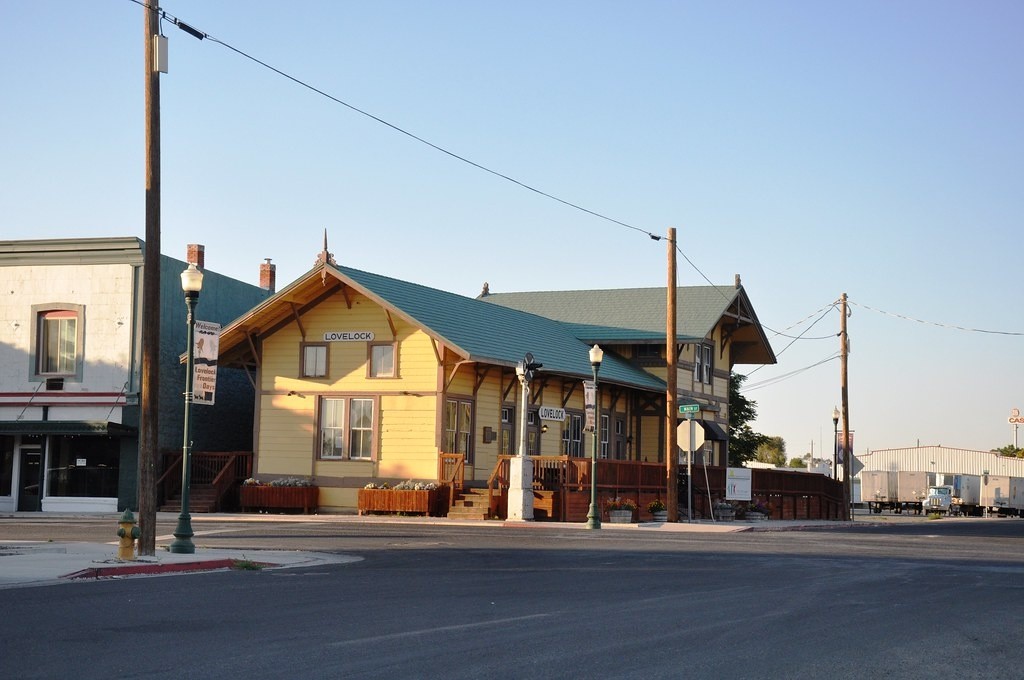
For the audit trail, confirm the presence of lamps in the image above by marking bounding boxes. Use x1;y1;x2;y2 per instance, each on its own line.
45;378;64;390
541;425;548;433
627;436;634;443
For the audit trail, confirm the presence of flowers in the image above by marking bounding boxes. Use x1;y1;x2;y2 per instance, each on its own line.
242;477;315;487
740;498;771;514
647;499;665;512
364;478;437;491
714;500;737;509
604;495;638;512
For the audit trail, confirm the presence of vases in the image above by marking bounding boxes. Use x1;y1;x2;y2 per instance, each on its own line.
714;510;736;521
357;488;439;517
653;511;668;522
239;485;319;516
744;511;763;523
609;510;633;523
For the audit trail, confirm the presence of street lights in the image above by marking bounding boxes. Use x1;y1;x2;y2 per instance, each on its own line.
830;406;840;479
584;343;604;530
169;263;206;552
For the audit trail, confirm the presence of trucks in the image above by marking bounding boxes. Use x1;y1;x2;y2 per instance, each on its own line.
920;486;963;515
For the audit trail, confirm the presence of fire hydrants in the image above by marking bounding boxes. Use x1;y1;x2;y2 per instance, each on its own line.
116;507;142;560
